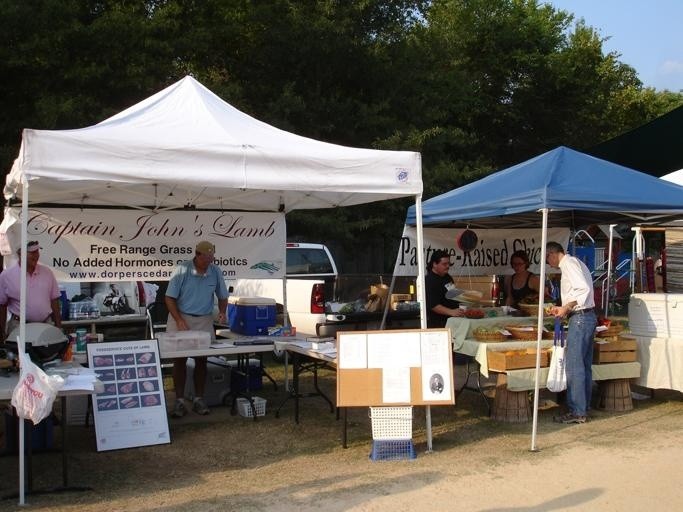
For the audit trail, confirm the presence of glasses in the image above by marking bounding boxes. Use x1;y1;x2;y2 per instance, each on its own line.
512;261;525;268
197;256;213;263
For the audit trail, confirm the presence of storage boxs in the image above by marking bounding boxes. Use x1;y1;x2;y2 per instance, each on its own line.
227;295;278;336
593;337;637;365
228;358;263;390
204;356;231;406
153;329;213;351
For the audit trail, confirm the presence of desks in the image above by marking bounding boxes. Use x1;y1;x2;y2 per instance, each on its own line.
276;339;343;424
216;326;316;393
61;313;150;341
629;334;683;400
1;361;95;492
72;337;275;421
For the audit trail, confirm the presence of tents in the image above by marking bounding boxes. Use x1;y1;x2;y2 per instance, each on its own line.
378;145;683;451
3;74;433;506
603;165;683;324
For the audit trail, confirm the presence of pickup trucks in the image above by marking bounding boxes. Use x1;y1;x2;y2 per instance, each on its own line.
211;239;415;362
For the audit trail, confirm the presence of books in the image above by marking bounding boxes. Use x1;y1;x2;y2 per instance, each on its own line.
305;335;334;343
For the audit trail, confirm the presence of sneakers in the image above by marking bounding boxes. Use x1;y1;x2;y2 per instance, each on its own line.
191;397;211;416
552;409;586;423
172;399;188;418
558;408;594;419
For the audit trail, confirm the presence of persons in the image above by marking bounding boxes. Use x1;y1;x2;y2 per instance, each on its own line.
0;238;62;345
546;241;598;423
165;240;229;417
505;250;548;307
432;378;443;393
424;250;465;328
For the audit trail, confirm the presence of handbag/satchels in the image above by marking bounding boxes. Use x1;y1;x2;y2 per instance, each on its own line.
546;315;568;393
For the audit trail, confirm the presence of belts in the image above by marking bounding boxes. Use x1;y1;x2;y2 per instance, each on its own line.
11;314;46;324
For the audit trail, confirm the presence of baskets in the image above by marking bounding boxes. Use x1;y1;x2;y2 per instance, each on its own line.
517;302;557;317
593;337;637;352
543;331;568;340
472;328;509;342
596;320;624;337
504;325;538;340
594;351;637;365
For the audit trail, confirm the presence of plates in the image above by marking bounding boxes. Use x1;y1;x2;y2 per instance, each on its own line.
464;313;484;319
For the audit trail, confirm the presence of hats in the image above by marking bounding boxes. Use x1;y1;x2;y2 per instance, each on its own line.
15;239;40;252
196;241;216;258
